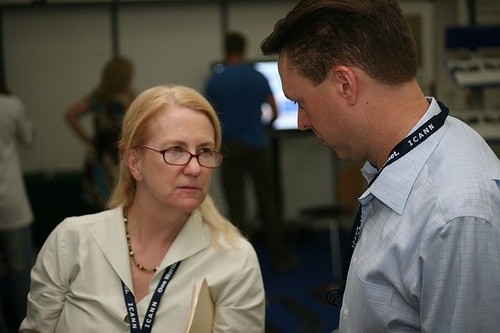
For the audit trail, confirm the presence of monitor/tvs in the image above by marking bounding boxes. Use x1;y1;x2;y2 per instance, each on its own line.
251;60;310;133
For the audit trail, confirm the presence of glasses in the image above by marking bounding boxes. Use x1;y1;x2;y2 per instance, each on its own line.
131;144;225;169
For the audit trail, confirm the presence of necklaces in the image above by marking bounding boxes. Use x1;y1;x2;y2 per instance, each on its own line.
124;206;159;272
121;260;181;332
324;101;450;305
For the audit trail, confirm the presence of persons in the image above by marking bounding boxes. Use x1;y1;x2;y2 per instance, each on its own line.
63;55;141;212
258;0;499;333
18;84;265;332
0;31;38;333
200;32;282;250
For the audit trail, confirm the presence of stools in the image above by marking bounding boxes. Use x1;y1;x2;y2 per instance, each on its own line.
299;205;355;302
